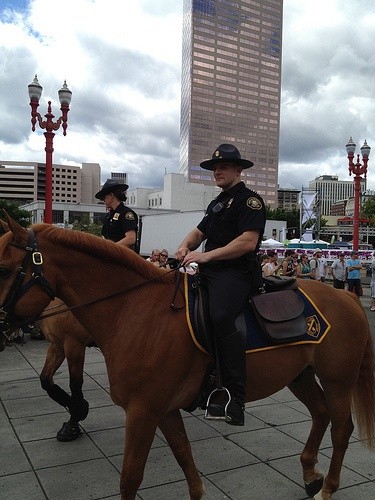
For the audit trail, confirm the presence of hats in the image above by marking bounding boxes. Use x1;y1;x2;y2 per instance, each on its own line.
94;179;129;200
315;248;322;253
350;250;358;255
198;144;256;171
339;253;344;255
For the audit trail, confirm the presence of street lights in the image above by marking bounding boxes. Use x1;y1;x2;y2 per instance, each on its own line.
28;73;72;226
345;135;371;258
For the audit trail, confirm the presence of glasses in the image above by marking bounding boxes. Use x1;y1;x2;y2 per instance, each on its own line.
292;257;298;259
161;253;169;258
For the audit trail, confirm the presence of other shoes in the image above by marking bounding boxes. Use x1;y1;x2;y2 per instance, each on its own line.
371;305;375;311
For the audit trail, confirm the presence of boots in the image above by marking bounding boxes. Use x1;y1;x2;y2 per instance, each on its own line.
208;330;246;427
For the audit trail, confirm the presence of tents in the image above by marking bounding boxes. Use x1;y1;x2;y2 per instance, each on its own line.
261;238;284;247
328;240;352;247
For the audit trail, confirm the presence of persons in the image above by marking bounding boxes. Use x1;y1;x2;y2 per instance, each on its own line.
12;325;44;344
94;179;139;252
147;248;375;311
176;144;266;425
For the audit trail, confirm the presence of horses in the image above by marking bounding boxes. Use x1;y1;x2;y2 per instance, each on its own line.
0;208;375;500
